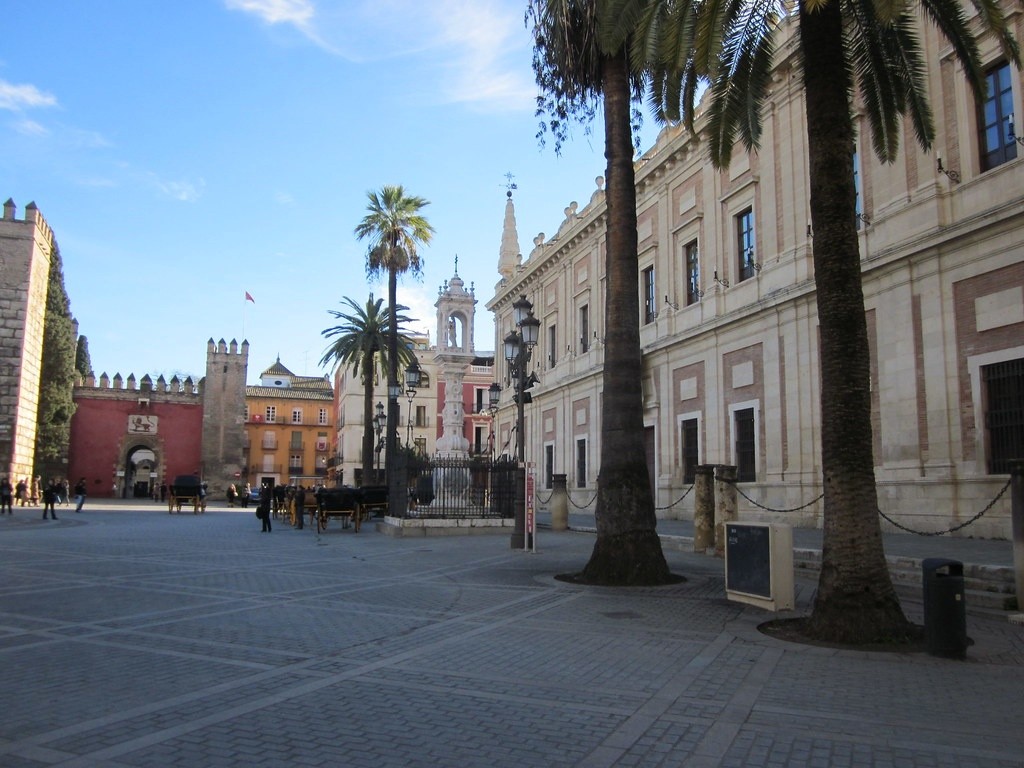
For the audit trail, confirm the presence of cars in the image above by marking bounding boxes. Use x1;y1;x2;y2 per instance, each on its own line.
248;487;262;504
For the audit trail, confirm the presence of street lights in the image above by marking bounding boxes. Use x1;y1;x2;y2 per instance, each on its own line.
370;400;387;485
486;382;503;463
403;360;422;452
500;293;540;548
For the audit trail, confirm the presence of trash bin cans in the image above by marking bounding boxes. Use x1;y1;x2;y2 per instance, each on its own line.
923;557;967;657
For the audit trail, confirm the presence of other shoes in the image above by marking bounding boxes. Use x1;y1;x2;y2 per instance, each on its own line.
261;529;267;532
295;527;303;529
268;528;272;532
228;505;231;508
231;505;233;508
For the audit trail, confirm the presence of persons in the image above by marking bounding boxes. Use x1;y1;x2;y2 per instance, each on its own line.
43;480;59;520
242;482;252;508
15;474;42;507
0;478;14;513
55;479;70;506
226;484;237;507
448;315;458;346
160;483;167;502
259;482;271;532
74;477;87;513
199;483;208;512
295;485;305;530
153;483;160;503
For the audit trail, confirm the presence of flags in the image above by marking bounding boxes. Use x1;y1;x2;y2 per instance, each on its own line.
246;292;254;302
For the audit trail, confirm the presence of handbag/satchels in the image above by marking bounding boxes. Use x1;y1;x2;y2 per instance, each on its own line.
255;507;261;520
